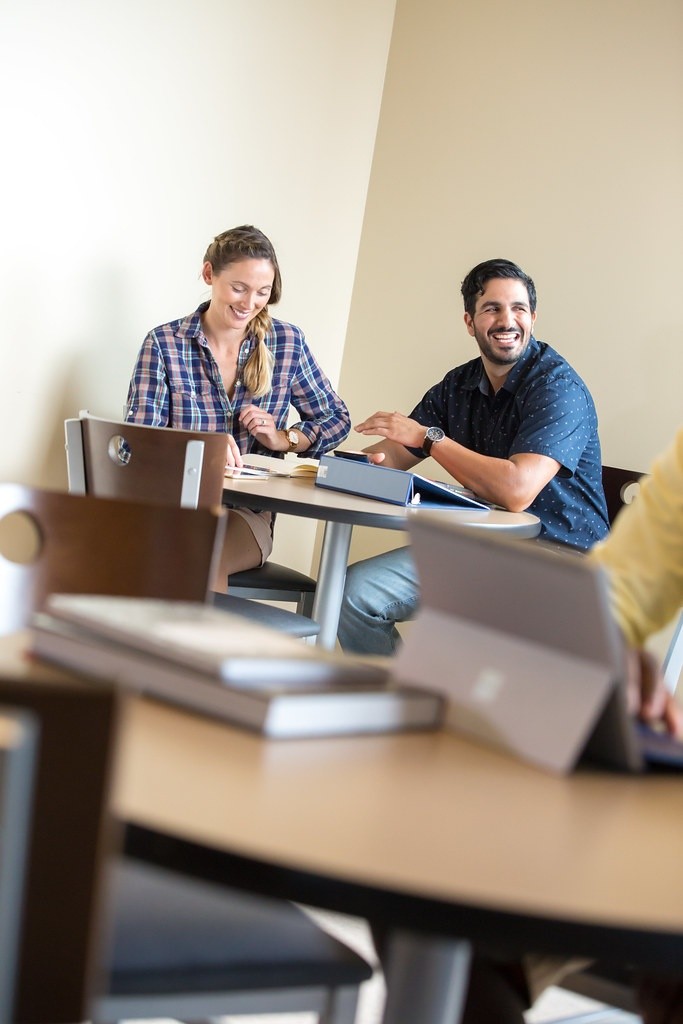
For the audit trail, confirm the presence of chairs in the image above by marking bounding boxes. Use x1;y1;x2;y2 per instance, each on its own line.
65;413;319;638
123;403;318;620
0;480;221;631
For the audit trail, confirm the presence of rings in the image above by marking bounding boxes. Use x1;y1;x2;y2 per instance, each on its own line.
262;420;266;425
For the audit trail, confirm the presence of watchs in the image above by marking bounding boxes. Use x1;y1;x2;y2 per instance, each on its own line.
283;430;299;452
422;427;445;456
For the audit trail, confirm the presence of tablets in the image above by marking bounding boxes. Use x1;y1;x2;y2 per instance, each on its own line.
408;511;641;775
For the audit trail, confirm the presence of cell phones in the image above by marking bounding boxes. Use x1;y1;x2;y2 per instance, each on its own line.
226;465;270;475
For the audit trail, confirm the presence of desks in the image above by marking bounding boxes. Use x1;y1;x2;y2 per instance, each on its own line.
221;475;542;652
1;626;683;1024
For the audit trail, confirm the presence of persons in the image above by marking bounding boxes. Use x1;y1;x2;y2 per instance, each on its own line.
457;423;682;1024
123;225;352;594
337;259;610;658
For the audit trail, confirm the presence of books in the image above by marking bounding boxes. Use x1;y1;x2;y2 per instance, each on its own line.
21;593;448;740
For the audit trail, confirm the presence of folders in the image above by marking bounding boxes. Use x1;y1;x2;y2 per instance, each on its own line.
315;453;498;511
